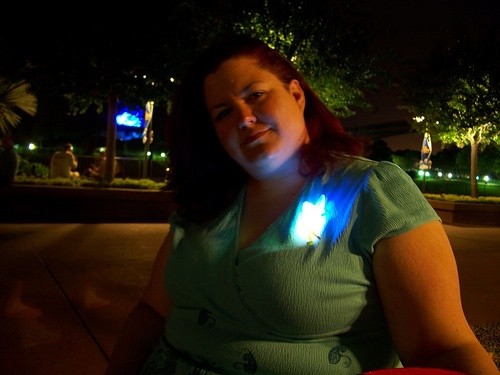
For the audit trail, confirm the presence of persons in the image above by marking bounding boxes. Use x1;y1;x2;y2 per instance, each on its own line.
50;144;80;179
103;34;500;375
89;148;120;178
0;133;19;186
